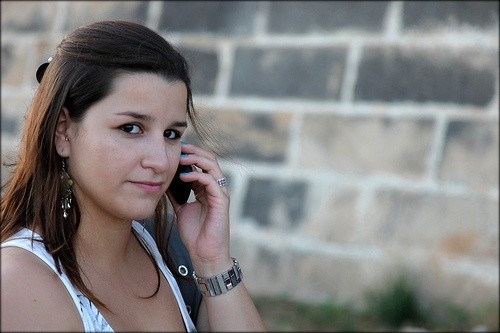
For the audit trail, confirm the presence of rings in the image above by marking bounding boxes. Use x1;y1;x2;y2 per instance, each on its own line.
215;178;228;186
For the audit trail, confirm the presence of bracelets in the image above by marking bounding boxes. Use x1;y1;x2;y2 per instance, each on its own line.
191;258;245;296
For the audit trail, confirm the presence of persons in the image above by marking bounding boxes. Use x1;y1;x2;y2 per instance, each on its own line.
2;21;265;333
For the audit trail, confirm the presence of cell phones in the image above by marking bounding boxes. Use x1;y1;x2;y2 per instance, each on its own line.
169;151;193;205
